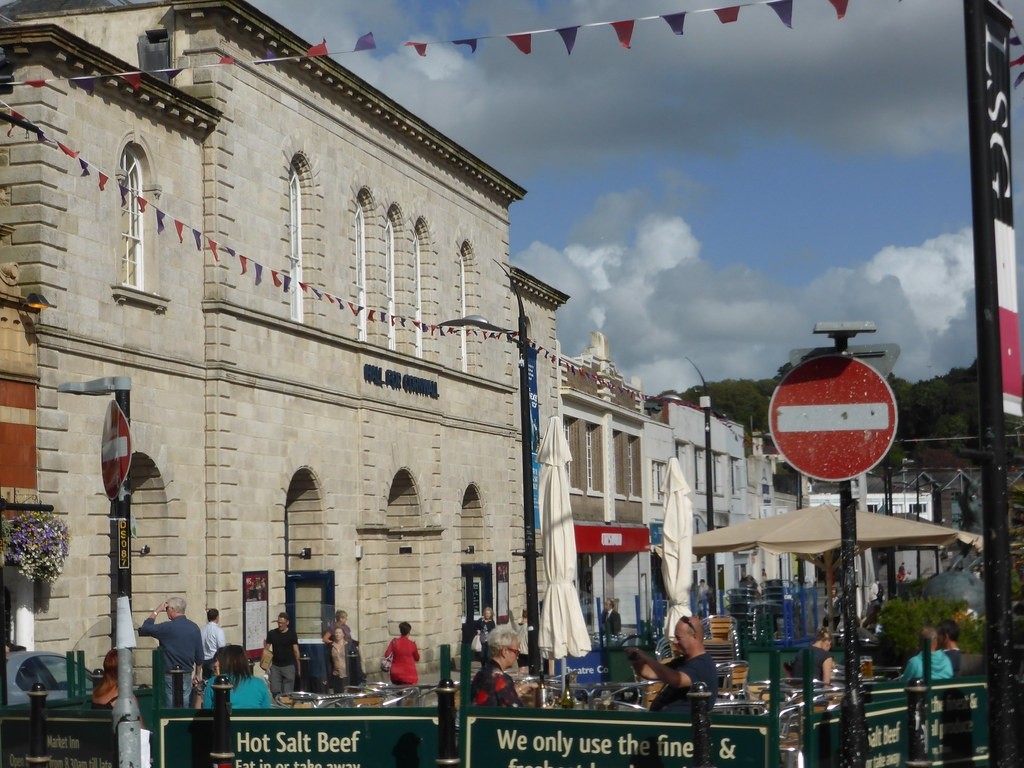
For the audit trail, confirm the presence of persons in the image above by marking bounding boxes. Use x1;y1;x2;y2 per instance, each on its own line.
321;611;367;707
900;599;978;684
822;586;842;632
193;645;273;708
199;608;226;676
477;606;496;668
90;649;144;729
385;622;420;706
471;624;523;707
861;578;884;634
601;599;621;636
789;628;834;690
625;616;718;714
898;560;912;580
137;597;203;709
264;612;301;707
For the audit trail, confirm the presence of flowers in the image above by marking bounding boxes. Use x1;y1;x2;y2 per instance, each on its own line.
2;511;72;594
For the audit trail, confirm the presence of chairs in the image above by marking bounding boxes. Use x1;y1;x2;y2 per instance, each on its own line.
273;578;905;768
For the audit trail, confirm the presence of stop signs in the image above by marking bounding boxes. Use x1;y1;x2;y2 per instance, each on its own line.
99;398;133;500
767;355;899;482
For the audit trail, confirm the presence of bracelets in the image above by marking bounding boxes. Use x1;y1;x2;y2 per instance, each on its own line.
152;611;158;615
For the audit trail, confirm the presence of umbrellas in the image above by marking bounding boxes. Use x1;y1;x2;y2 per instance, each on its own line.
537;411;592;706
658;455;694;644
644;499;1024;645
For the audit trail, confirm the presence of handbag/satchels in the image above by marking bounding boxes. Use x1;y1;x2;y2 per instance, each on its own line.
471;630;482;652
380;638;394;671
261;648;273;671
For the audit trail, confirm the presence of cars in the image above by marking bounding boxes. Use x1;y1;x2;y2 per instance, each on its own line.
6;649;95;707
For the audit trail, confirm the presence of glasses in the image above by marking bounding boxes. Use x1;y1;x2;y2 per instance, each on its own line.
504;647;521;657
681;615;697;635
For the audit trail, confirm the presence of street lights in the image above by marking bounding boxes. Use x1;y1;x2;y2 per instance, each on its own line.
433;254;546;676
55;374;144;768
809;320;882;768
656;354;720;617
898;457;916;519
